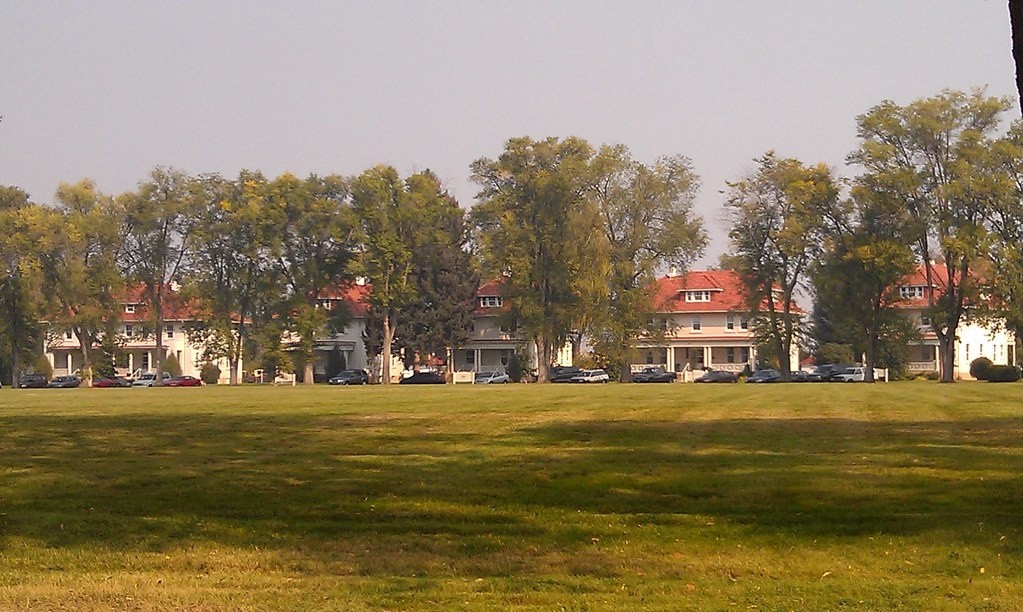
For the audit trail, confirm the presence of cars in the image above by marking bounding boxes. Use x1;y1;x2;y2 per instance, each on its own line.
398;373;445;383
474;371;510;384
45;375;79;389
744;369;782;382
92;377;115;387
693;370;738;384
162;376;201;387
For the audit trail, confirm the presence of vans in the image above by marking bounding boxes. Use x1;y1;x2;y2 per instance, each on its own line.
132;372;171;387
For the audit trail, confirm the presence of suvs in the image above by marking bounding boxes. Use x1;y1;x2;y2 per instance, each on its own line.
19;374;47;388
831;367;879;382
328;369;368;385
806;364;846;382
551;368;579;382
570;369;609;383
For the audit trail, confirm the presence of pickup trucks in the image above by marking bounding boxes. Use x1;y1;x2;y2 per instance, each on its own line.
632;367;677;383
790;371;821;381
